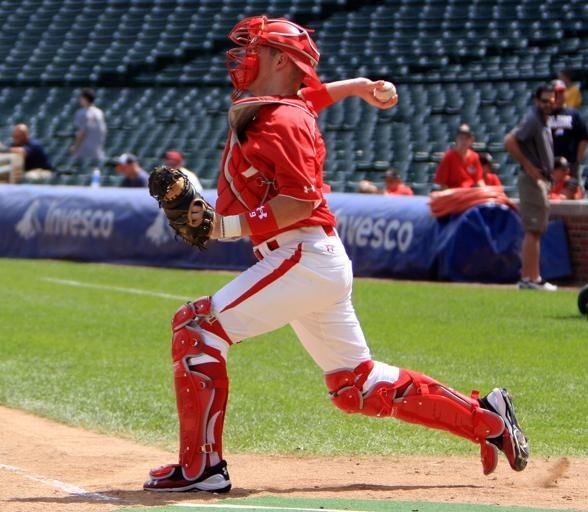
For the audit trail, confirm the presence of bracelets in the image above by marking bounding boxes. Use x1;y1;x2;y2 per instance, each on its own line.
219;212;244;243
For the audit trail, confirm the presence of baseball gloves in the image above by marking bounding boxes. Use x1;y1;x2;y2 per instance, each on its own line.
148;165;214;250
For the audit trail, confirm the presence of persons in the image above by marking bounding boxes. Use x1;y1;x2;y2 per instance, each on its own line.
428;121;487;194
139;13;531;496
544;77;587;195
478;150;511;199
382;164;416;198
113;150;151;188
557;69;584;112
502;84;560;294
546;155;588;201
162;148;203;192
67;90;108;187
0;122;61;184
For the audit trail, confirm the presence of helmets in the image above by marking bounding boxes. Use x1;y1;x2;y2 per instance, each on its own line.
226;15;320;101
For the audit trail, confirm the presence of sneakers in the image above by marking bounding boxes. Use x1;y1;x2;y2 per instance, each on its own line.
519;278;556;291
143;460;231;492
478;387;528;472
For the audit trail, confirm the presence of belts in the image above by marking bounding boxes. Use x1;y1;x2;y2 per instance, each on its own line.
253;226;336;260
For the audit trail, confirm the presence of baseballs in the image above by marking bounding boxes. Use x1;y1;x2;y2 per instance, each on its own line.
374;81;396;103
